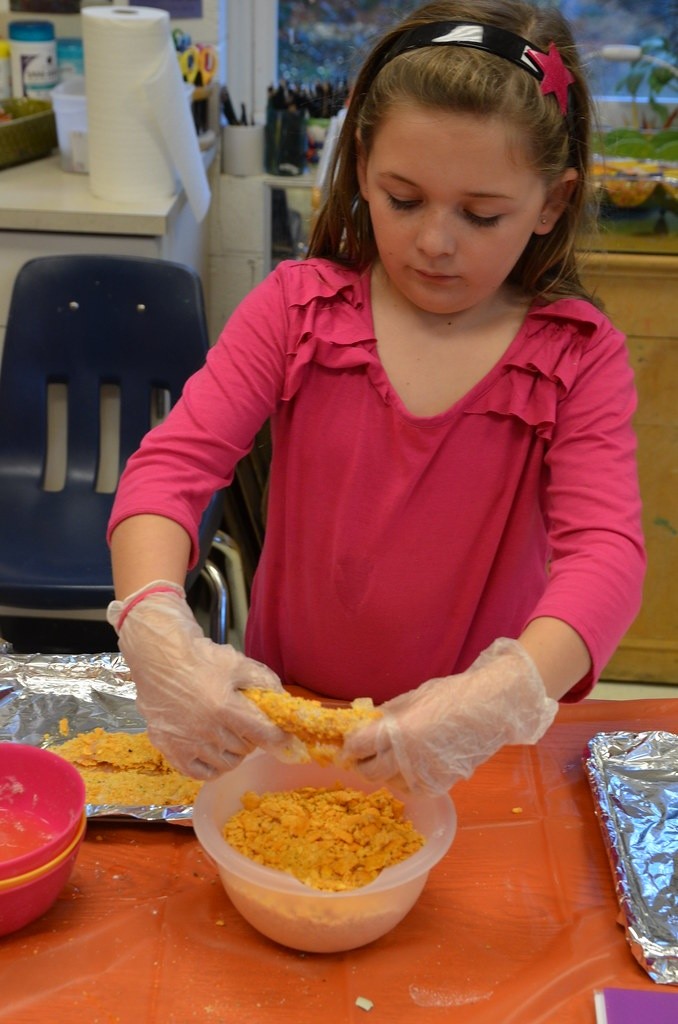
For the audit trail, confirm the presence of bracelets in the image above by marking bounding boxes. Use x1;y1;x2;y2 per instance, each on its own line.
116;587;181;636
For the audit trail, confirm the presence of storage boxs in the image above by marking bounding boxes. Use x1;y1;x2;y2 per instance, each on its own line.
50;76;195;176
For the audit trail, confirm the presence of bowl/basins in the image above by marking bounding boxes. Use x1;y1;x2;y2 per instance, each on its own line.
192;747;457;953
0;743;87;936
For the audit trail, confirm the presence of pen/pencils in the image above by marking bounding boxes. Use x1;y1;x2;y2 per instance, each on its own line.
218;87;256;127
265;78;345;165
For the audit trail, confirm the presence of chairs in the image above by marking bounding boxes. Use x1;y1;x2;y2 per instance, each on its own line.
0;255;248;658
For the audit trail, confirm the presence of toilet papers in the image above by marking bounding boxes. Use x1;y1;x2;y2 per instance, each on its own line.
80;5;212;224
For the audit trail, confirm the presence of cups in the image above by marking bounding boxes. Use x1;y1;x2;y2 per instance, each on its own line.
267;119;306;173
307;119;333;164
221;124;263;177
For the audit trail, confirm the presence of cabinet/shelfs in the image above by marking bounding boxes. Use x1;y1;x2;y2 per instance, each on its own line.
547;256;678;690
0;147;224;347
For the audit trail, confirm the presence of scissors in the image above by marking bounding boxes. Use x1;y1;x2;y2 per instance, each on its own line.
182;46;218;87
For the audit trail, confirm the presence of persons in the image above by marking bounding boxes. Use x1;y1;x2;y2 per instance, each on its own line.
109;0;646;803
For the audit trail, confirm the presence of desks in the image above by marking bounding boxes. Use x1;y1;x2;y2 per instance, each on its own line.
0;700;678;1024
